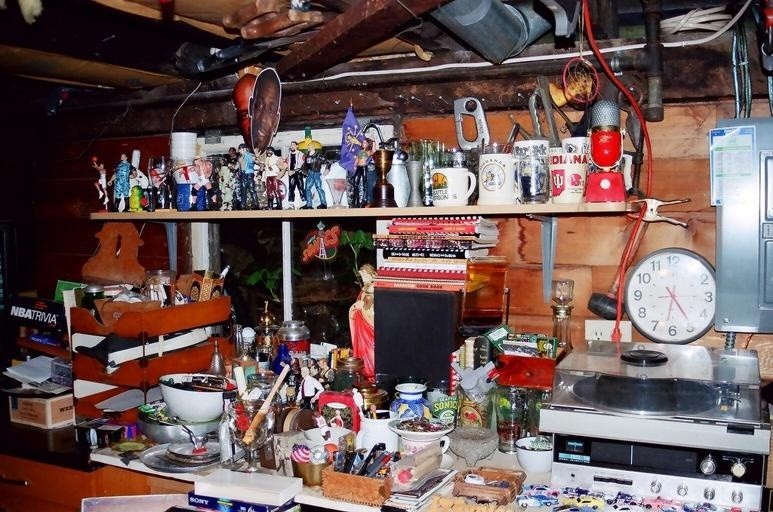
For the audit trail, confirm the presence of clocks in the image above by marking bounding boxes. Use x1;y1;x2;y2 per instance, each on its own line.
624;246;717;344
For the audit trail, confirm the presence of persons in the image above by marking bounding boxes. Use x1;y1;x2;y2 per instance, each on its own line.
347;262;374;384
284;355;376;430
349;295;373;314
85;137;375;211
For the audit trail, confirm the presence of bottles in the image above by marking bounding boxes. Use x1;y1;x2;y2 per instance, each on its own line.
79;269;554;487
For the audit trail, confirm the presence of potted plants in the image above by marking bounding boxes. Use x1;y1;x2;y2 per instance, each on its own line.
515;436;554;472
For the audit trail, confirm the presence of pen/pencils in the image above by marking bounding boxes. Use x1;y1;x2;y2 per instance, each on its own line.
343;443;394;478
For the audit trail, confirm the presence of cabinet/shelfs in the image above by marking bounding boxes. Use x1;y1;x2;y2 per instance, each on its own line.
72;294;234;426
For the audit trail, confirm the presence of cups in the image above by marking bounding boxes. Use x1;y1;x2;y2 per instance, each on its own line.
119;129;630;212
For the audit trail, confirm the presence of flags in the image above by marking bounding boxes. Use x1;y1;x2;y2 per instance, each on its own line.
338;107;365;176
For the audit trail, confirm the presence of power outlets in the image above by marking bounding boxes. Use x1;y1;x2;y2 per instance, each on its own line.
584;320;632;343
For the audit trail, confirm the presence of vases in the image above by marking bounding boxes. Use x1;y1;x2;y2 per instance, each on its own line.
389;383;431;418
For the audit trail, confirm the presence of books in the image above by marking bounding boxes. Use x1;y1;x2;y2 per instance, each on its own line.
187;469;303;512
382;468;458;512
370;214;499;293
449;333;491;395
